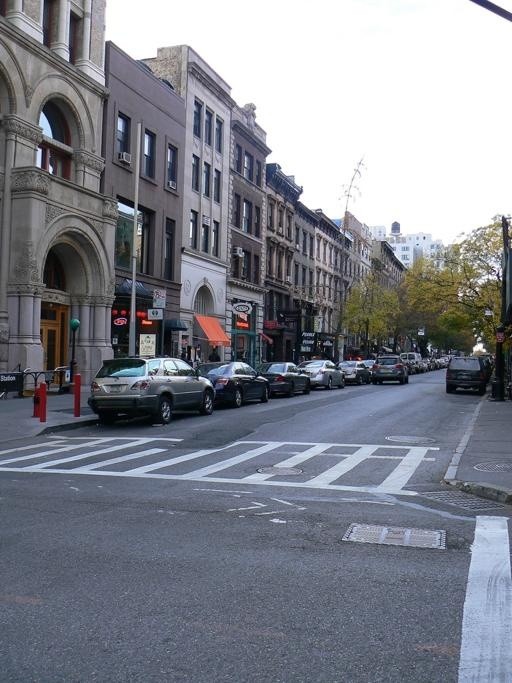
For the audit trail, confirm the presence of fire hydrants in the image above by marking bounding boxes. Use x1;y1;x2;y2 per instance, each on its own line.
34;388;40;416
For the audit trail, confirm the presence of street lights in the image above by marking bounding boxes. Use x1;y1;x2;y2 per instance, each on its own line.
70;319;80;382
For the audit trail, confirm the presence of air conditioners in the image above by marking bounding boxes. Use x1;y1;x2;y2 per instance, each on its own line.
296;244;300;250
286;276;292;284
233;247;242;257
168;181;176;190
118;152;131;164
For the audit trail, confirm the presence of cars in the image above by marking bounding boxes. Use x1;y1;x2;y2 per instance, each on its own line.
55;366;70;382
